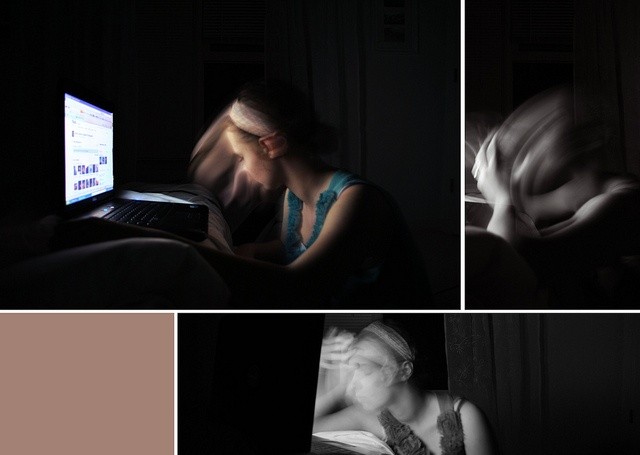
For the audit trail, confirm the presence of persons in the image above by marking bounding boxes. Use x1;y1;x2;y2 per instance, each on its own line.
197;83;383;302
313;322;501;454
468;93;637;309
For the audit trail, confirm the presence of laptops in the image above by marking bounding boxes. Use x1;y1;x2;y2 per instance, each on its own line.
56;91;209;243
